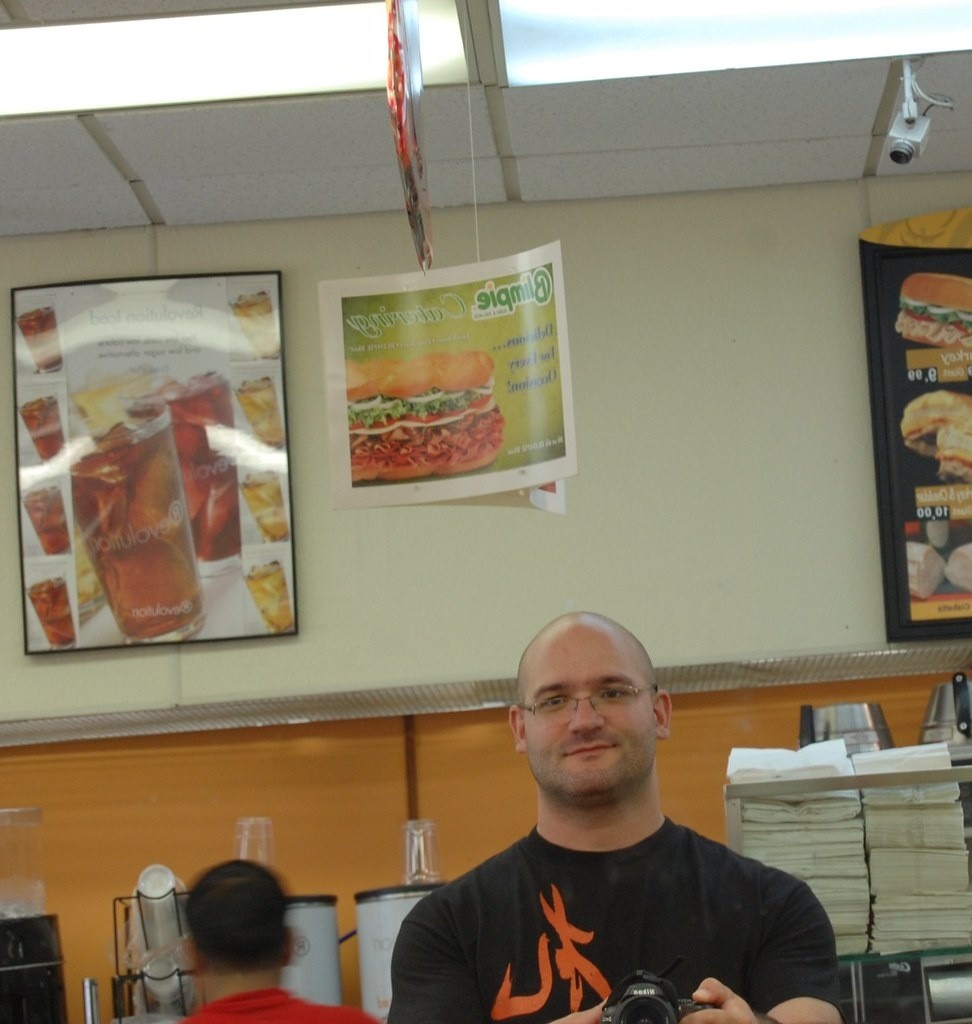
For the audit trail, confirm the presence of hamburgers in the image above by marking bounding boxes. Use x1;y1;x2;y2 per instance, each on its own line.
896;272;971;352
345;352;504;482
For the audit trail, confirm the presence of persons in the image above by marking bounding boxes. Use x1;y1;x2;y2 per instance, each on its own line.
174;859;385;1024
386;612;844;1024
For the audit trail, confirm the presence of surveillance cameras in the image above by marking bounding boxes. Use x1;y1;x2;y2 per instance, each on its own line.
888;112;931;165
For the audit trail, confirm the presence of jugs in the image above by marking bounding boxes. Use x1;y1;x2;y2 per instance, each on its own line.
0;804;47;921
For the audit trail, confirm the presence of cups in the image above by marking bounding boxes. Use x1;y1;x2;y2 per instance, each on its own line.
12;298;81;654
65;364;245;643
229;286;299;637
234;816;275;872
401;817;445;888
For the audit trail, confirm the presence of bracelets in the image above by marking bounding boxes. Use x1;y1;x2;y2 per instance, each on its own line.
754;1012;782;1024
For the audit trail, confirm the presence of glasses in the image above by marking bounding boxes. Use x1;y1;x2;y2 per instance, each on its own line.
513;682;659;727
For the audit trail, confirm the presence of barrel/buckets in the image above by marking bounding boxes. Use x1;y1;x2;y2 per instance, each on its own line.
352;881;456;1024
276;891;344;1007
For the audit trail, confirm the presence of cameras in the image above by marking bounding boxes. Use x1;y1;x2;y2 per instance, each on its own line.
601;979;715;1024
